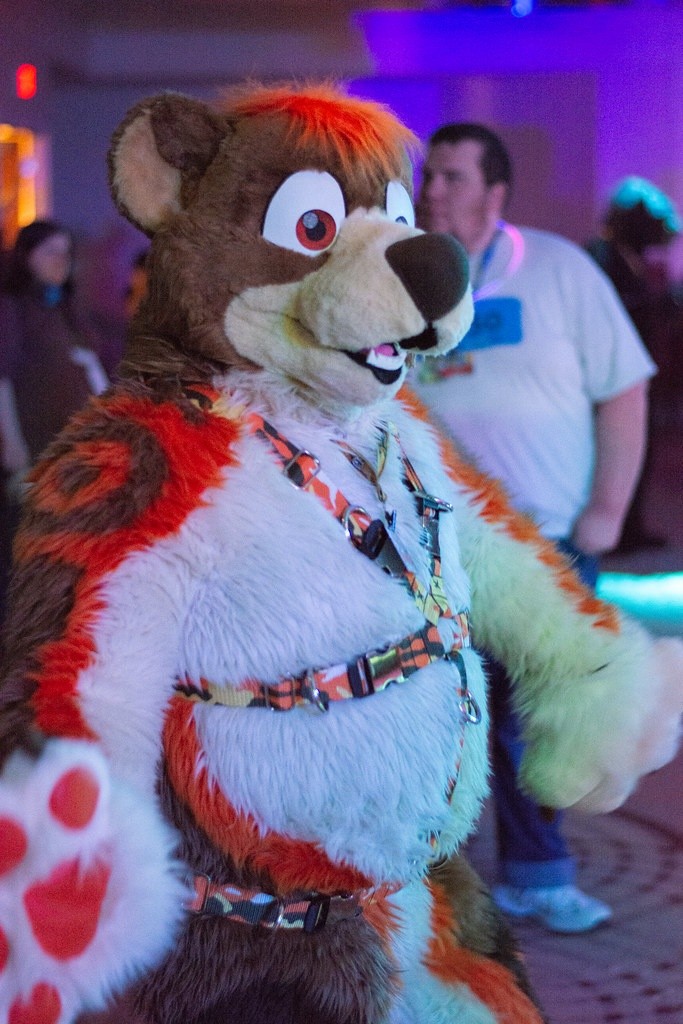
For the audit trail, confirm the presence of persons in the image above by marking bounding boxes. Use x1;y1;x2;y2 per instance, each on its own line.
579;182;680;548
3;218;112;498
409;121;655;931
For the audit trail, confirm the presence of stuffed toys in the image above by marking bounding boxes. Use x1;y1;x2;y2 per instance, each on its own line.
1;89;683;1024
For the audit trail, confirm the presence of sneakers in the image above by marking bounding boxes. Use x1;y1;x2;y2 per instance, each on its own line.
494;879;613;933
